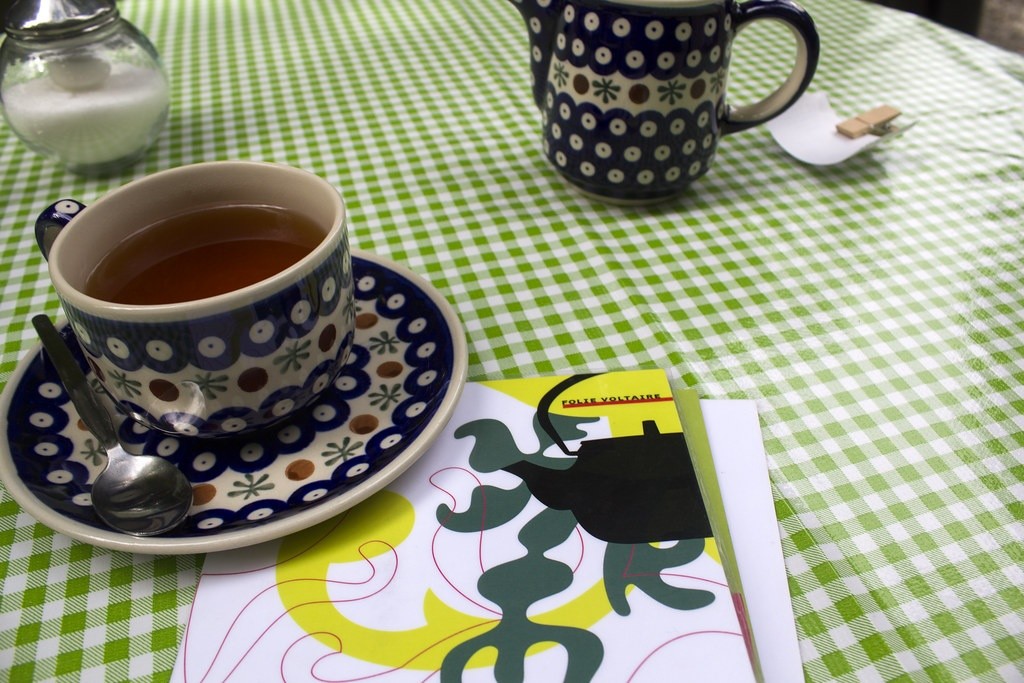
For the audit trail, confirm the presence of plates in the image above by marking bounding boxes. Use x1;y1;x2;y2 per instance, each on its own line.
0;246;470;553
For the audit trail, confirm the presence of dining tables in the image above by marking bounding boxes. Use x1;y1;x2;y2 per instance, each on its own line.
1;0;1024;683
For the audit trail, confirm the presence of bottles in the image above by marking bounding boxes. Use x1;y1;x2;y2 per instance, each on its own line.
0;0;170;178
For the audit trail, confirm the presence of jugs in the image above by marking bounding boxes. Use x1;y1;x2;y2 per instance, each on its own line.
501;0;820;206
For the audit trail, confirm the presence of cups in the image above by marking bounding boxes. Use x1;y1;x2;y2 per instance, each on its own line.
34;161;356;437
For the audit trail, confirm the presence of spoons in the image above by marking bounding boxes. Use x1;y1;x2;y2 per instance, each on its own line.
32;315;193;537
